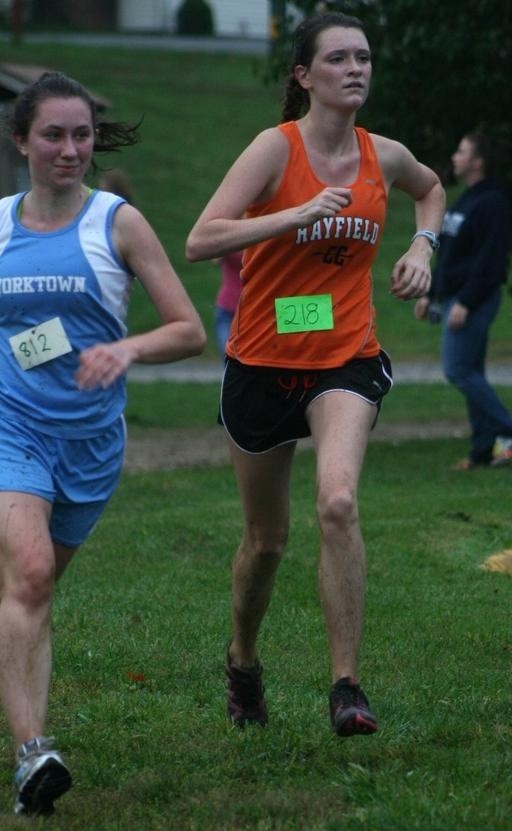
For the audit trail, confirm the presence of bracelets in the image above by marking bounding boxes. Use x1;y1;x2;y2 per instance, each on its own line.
413;231;440;249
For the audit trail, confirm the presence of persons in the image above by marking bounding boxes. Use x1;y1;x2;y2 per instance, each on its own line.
213;211;247;427
183;11;450;738
98;166;130;203
1;64;209;816
413;132;512;473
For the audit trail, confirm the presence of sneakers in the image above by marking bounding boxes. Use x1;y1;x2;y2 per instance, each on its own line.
488;437;512;469
225;638;269;728
446;454;487;470
11;736;74;817
327;676;378;737
